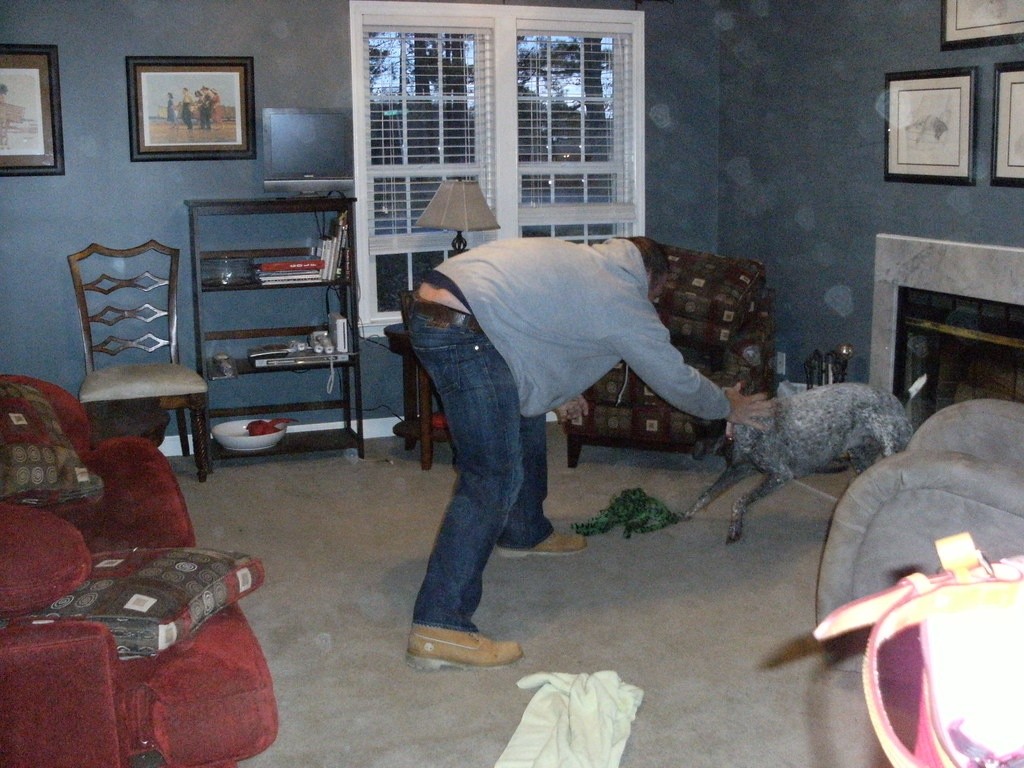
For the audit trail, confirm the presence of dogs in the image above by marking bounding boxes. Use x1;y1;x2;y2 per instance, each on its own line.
684;359;929;545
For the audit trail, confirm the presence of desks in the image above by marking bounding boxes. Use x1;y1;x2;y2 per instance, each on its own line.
385;323;450;470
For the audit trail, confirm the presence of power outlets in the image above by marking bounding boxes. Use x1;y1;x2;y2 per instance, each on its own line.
767;351;786;377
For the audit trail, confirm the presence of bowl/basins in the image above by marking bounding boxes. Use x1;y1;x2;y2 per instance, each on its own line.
211;418;287;451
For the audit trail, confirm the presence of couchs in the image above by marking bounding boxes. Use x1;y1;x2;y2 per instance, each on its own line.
0;375;275;768
563;236;777;468
814;397;1024;675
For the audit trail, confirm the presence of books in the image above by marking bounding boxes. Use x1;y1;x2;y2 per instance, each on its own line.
255;218;348;287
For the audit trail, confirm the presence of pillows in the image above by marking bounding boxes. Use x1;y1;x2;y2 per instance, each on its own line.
0;546;264;659
0;500;93;618
0;382;105;513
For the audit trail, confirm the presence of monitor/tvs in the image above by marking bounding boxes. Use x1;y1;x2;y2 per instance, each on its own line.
261;108;354;198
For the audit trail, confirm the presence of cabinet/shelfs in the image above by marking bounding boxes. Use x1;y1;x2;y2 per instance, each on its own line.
184;197;365;476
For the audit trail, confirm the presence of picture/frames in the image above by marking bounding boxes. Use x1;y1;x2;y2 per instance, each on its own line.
939;0;1024;53
0;43;66;178
989;60;1024;188
883;65;979;185
125;55;256;162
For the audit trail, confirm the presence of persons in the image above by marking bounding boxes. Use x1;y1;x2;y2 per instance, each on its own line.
167;86;225;130
399;236;774;672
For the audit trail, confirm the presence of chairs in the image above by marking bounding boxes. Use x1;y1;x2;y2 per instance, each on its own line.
67;240;210;485
399;291;418;323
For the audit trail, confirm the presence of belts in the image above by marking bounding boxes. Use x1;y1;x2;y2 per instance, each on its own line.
412;299;480;330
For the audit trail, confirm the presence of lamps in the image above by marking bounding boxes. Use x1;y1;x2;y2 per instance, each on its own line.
414;180;502;257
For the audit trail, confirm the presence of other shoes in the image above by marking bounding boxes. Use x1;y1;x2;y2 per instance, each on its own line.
495;530;587;558
405;630;523;672
